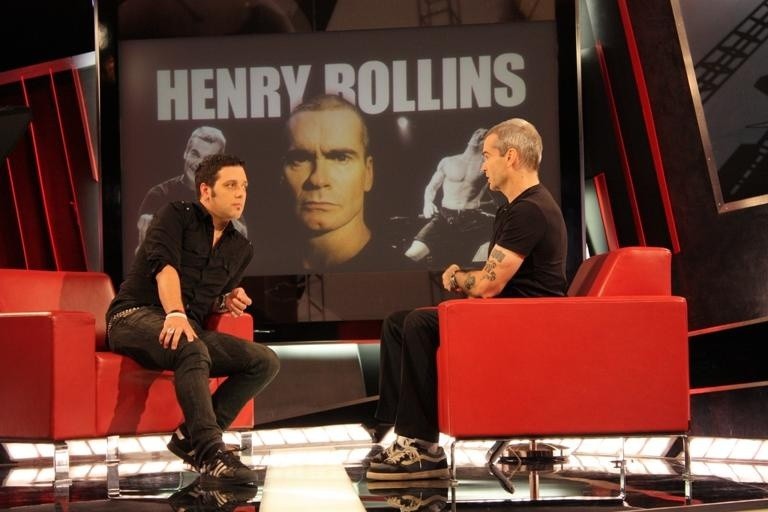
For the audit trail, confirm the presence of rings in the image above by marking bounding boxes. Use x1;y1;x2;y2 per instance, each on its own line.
166;326;175;335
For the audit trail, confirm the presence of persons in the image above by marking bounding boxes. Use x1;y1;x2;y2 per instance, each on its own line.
104;153;286;491
402;124;499;266
134;124;252;258
362;117;570;488
158;483;260;512
243;92;432;269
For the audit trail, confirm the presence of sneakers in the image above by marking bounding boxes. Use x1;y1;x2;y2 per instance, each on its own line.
167;475;257;512
366;441;449;480
167;423;199;473
199;445;258;485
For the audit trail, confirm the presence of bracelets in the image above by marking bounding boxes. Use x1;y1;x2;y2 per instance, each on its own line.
216;293;228;314
163;309;188;319
450;269;463;290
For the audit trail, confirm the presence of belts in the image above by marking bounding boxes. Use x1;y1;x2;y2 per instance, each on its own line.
107;307;140;335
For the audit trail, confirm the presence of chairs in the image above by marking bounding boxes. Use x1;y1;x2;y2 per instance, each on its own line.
416;246;692;486
0;270;253;483
